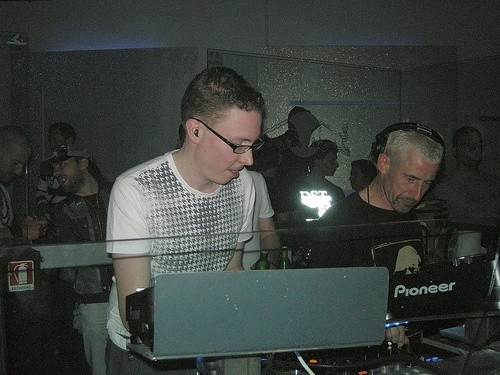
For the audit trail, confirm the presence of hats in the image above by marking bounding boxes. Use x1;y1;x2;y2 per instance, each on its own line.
40;143;92;182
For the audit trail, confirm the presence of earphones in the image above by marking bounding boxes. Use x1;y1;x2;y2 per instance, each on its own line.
194;129;198;137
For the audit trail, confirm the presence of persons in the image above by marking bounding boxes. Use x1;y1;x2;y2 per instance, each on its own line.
296;122;447;346
0;122;115;375
244;134;378;269
431;127;500;214
105;66;265;375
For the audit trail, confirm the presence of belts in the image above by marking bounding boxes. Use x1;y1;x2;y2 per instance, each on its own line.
75;293;109;304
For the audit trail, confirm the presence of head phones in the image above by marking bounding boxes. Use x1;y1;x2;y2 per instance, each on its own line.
370;122;446;186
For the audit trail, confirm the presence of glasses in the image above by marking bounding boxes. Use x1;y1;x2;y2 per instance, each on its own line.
189;117;265;154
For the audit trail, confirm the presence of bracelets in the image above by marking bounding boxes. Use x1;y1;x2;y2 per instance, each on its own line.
8;224;23;237
